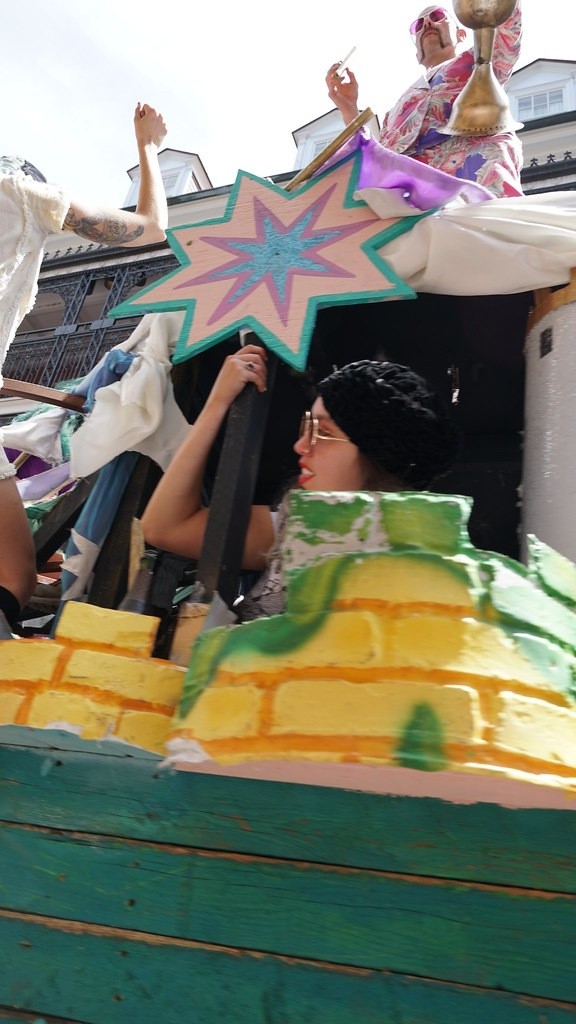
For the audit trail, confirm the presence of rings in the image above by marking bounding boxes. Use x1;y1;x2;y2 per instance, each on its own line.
248;362;255;368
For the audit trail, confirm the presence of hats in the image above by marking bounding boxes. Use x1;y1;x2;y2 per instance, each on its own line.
315;359;443;484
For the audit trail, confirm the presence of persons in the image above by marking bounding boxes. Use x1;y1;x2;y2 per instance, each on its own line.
325;0;526;200
0;101;168;642
138;344;440;622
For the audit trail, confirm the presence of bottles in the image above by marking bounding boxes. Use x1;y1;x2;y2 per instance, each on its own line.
119;549;160;614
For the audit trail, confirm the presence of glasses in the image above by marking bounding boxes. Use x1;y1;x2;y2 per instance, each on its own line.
299;411;350;446
409;7;456;46
